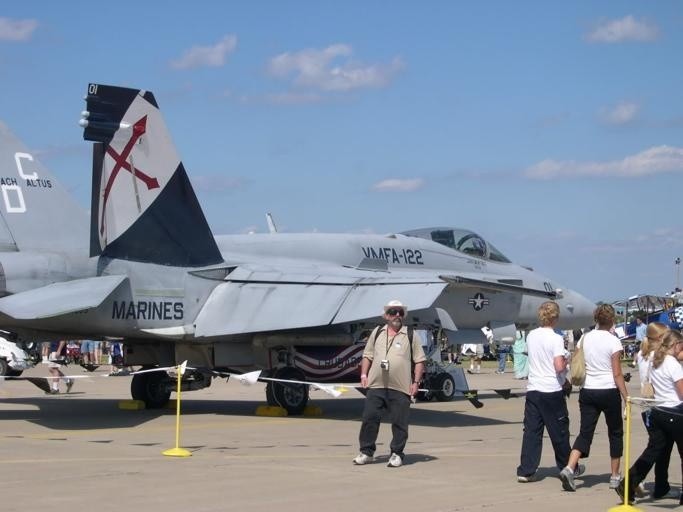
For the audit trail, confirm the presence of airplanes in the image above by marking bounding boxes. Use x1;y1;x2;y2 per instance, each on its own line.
0;82;598;415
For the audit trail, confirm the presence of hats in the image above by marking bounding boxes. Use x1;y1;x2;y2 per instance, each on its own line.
383;300;408;320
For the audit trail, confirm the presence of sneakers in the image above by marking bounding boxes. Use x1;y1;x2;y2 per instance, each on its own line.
574;464;585;479
352;451;374;465
387;452;402;468
661;487;680;499
615;485;636;505
559;466;578;491
465;369;504;374
517;473;539;482
628;363;635;367
609;475;622;488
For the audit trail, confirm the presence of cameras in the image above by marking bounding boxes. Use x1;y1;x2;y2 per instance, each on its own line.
381;360;389;371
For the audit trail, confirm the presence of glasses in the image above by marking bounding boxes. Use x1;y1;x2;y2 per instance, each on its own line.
386;308;405;317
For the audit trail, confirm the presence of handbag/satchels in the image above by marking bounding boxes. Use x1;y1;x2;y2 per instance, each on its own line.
570;345;586;386
643;382;655;403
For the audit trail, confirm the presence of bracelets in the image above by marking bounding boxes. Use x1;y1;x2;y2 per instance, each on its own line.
413;381;420;386
360;374;367;378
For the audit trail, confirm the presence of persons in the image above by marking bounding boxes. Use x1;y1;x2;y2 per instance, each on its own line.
516;301;586;483
34;338;128;395
613;329;682;504
637;320;683;496
558;304;631;491
352;299;426;468
447;315;647;379
